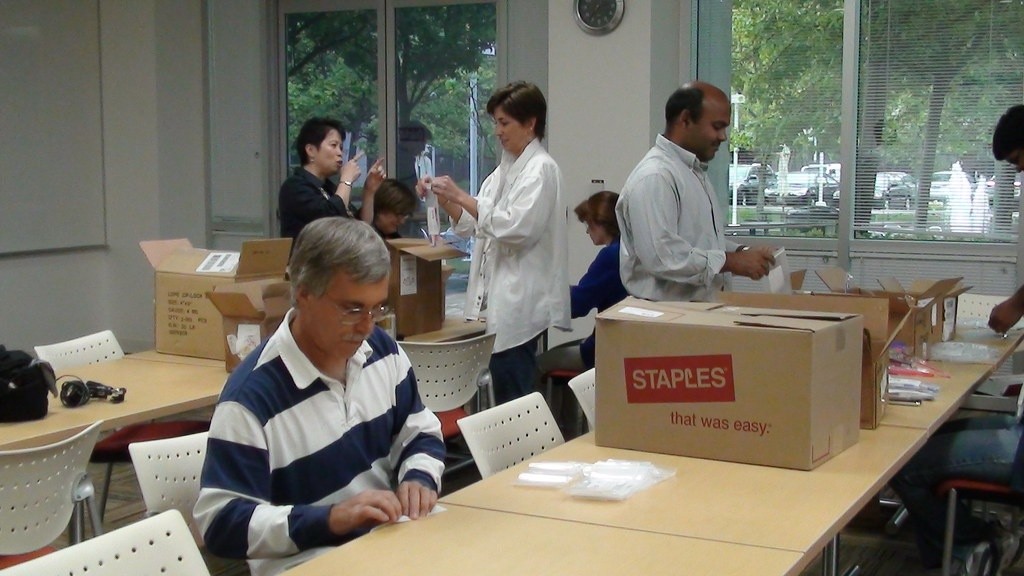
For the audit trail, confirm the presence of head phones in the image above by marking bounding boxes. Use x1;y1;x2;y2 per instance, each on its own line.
59;380;126;409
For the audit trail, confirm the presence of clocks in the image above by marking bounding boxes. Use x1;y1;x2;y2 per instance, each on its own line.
573;0;625;36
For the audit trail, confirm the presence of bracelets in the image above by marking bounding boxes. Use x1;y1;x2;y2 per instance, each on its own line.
741;246;751;252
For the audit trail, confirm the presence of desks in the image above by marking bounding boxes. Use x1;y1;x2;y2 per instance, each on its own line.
275;327;1024;576
0;317;488;547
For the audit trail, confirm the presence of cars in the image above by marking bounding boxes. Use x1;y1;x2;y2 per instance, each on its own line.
726;162;1022;209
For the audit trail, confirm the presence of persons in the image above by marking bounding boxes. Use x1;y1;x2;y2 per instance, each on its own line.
886;414;1024;576
194;216;445;576
987;103;1024;333
415;79;573;402
276;116;388;238
353;178;416;241
534;190;628;380
614;81;778;302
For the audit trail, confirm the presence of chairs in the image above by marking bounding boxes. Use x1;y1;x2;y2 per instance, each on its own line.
33;328;212;523
128;430;211;548
543;368;584;438
455;391;565;479
0;509;212;576
939;477;1018;576
0;420;104;572
567;367;595;429
394;333;497;474
955;291;1024;331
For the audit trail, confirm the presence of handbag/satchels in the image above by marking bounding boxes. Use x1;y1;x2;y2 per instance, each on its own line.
0;345;48;422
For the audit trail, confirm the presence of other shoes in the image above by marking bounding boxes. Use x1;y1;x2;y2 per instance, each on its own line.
951;528;993;576
986;520;1024;576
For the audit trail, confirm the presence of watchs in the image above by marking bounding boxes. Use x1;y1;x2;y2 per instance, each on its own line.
339;180;353;188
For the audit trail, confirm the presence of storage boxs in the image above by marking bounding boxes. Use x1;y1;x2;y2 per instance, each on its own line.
137;238;472;374
594;266;974;472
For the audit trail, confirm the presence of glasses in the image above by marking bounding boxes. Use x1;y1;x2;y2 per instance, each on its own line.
391;212;411;219
323;293;389;324
